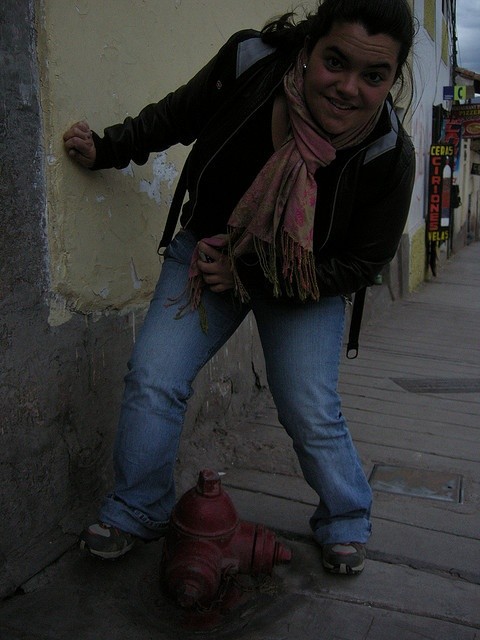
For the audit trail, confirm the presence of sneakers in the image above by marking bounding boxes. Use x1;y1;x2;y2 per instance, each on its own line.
77;520;161;558
321;540;368;575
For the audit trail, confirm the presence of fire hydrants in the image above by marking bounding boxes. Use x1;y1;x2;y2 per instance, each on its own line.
165;470;293;606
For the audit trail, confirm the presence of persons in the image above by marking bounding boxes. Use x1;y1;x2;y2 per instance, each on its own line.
65;2;417;574
